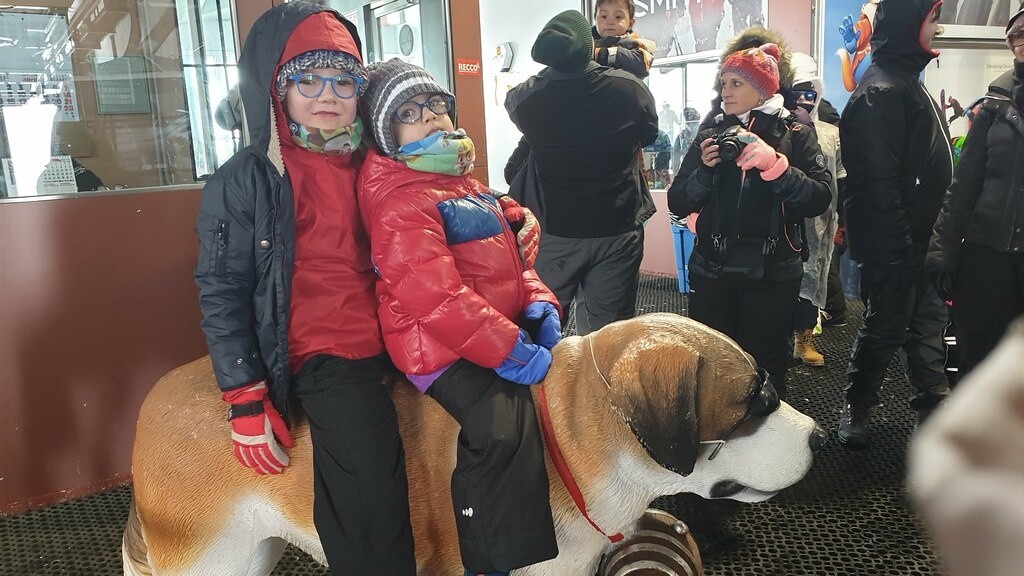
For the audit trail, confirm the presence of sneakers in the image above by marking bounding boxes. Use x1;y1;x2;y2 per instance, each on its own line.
820;308;849;326
837;399;870;448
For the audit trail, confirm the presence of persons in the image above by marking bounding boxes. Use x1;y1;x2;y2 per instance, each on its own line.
192;0;539;575
642;131;673;173
788;52;847;369
671;107;701;179
925;2;1024;391
839;0;954;445
591;0;658;189
356;57;559;576
504;9;658;334
658;101;681;145
667;26;833;402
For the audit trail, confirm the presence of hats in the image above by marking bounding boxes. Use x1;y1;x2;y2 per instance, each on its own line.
792;82;816;92
275;49;370;103
531;10;593;75
1005;12;1024;55
720;43;780;100
356;56;457;157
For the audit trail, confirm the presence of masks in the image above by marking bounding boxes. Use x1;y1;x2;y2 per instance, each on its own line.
796;104;814;113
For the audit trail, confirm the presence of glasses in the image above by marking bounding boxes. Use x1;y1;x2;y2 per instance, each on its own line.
1008;31;1024;47
97;184;127;191
286;73;363;99
792;91;816;101
392;93;454;124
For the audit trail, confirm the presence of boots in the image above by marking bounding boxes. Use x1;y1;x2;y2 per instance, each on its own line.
793;330;826;367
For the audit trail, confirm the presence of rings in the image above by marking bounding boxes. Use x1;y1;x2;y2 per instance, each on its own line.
705;156;710;161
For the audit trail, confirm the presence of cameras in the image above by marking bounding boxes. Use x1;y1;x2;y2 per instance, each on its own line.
706;124;748;161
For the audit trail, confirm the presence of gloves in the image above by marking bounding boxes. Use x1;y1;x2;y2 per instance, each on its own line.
928;270;953;303
525;301;562;350
886;236;919;295
734;131;790;181
493;330;552;385
686;212;699;235
499;196;541;270
222;380;294;476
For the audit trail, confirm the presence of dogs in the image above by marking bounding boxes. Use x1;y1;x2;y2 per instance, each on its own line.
122;311;826;576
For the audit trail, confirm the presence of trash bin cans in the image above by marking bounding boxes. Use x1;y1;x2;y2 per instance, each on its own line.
672;226;697;294
